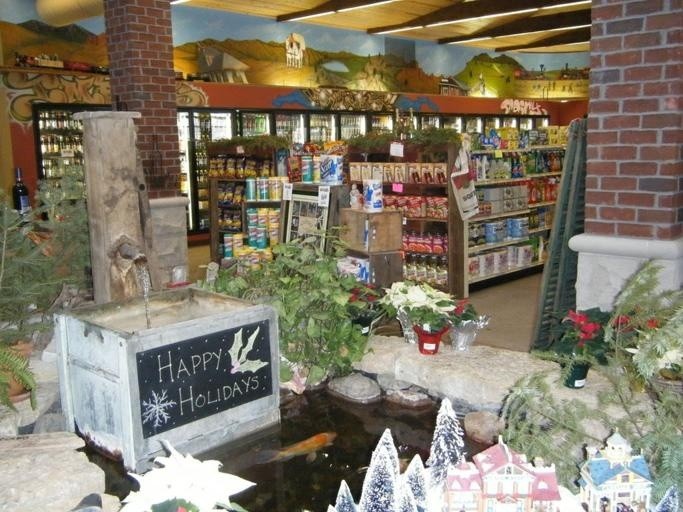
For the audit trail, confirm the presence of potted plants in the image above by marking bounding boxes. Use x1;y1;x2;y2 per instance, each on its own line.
0;165;91;414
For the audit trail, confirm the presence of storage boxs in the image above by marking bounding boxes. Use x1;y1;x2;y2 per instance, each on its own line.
346;247;406;289
339;208;402;250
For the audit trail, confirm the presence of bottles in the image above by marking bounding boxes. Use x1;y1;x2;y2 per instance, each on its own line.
13;167;29;210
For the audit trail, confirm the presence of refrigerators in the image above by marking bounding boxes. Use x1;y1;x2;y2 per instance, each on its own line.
35;114;551;249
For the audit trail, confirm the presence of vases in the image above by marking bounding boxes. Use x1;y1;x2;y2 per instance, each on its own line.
412;325;449;355
559;361;592;390
655;378;683;395
452;330;478;352
605;352;627;376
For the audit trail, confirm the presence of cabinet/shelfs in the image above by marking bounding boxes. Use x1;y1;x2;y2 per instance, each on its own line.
463;145;564;299
346;141;463;299
208;148;276;266
187;140;211;247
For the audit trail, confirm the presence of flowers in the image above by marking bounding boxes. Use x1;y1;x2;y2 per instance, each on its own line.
561;309;602;356
347;281;491;334
625;308;683;382
602;305;658;352
